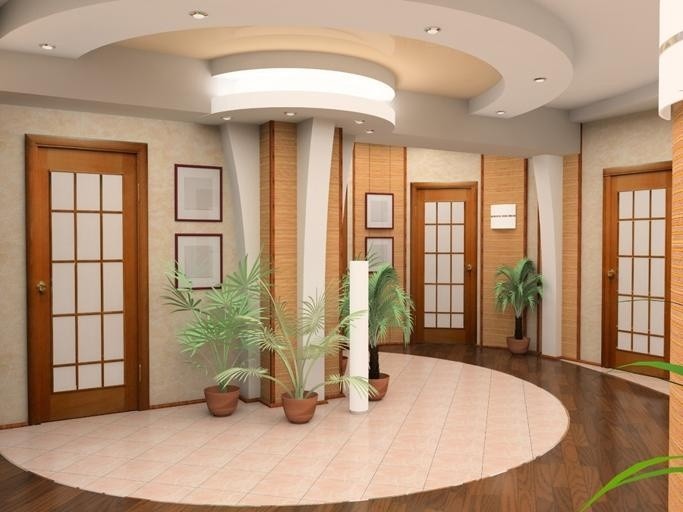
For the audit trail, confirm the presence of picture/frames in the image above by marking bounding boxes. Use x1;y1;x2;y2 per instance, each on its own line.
172;163;223;290
365;190;394;273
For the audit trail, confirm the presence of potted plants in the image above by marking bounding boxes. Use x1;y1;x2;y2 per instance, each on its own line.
493;255;549;355
